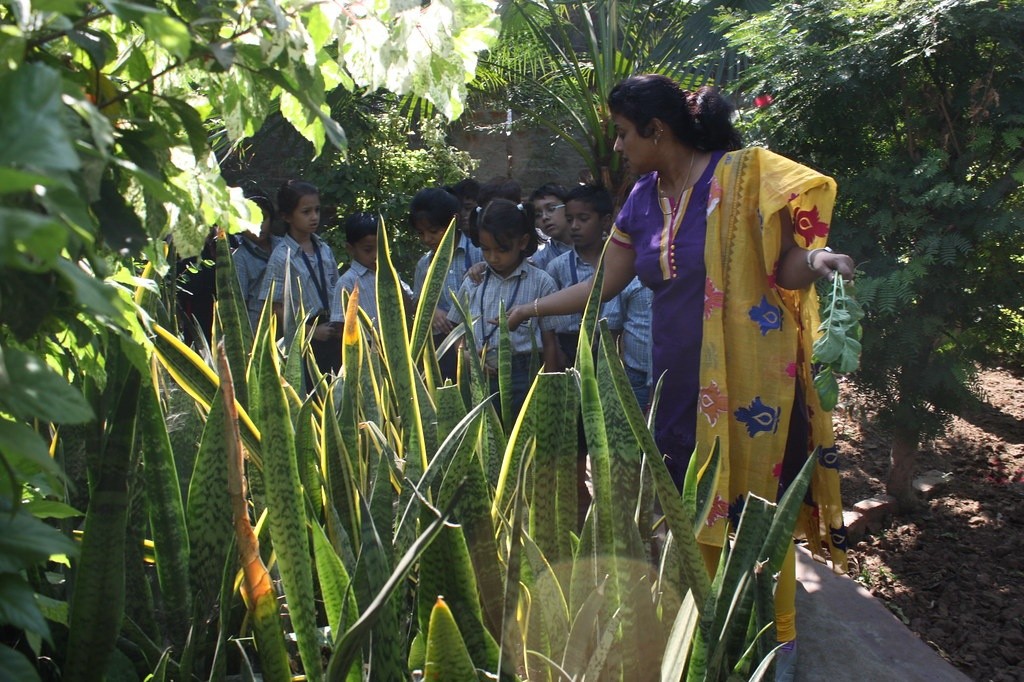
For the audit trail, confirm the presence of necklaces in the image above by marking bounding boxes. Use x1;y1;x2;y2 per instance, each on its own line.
658;152;694;215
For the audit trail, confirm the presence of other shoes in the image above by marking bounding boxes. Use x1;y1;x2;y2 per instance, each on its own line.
775;640;798;682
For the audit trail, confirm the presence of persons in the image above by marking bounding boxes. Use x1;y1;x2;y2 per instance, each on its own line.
171;177;654;502
487;74;854;682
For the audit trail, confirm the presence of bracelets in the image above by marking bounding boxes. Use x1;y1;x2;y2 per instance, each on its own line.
535;298;540;316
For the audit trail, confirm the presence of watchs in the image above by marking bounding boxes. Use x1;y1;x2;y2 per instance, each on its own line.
807;246;834;272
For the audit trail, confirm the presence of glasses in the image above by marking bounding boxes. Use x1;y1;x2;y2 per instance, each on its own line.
535;204;565;219
